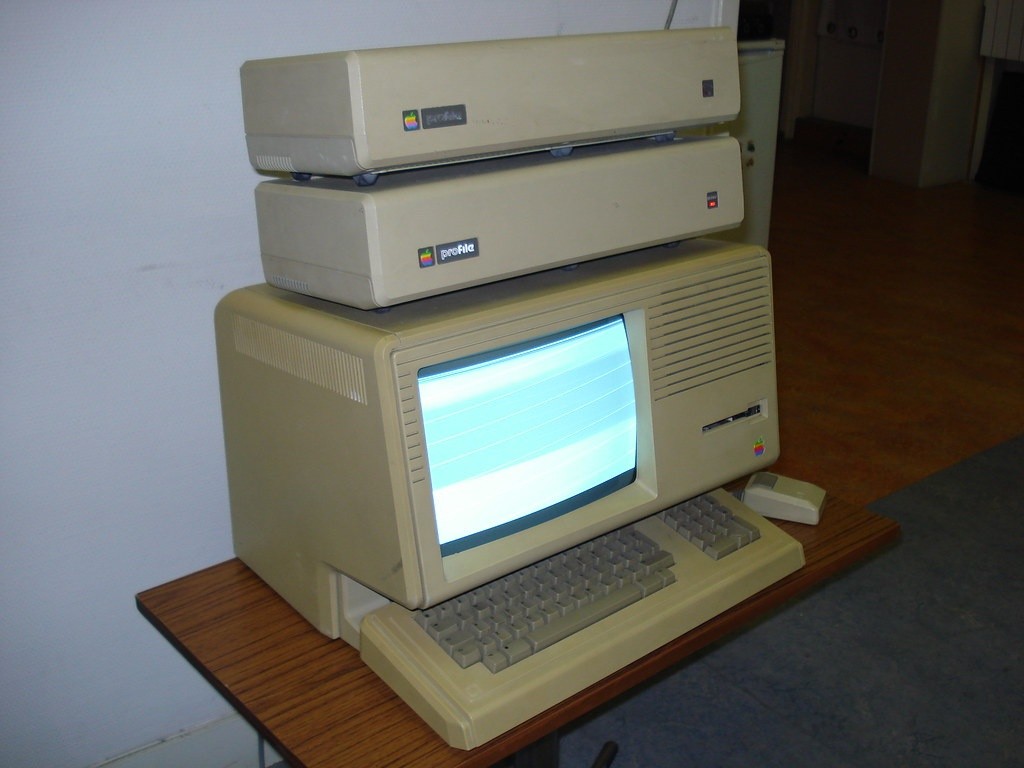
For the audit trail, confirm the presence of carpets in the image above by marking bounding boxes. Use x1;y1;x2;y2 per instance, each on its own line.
560;433;1024;768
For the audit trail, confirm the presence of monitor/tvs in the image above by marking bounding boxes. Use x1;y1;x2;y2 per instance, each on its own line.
214;236;780;649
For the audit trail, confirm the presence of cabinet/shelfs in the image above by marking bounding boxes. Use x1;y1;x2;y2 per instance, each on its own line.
699;39;786;248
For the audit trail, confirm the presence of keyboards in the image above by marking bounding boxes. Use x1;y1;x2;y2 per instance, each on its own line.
358;488;805;751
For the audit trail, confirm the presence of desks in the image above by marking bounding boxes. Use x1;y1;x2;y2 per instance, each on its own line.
135;471;902;768
